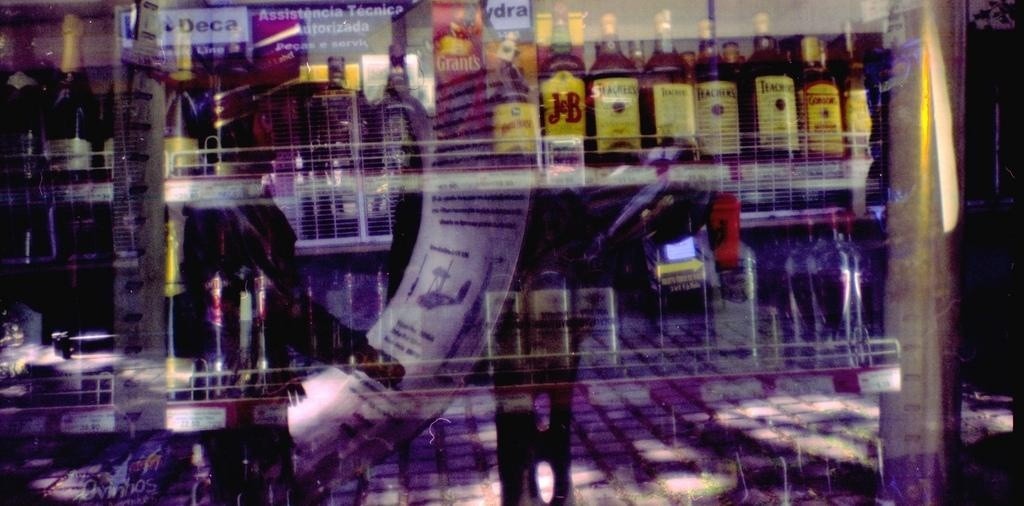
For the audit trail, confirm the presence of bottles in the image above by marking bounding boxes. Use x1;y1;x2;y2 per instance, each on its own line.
0;0;900;506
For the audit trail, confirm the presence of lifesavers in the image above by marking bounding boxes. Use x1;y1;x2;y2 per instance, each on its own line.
0;3;538;502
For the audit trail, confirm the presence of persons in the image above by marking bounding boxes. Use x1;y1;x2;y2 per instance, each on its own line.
170;80;412;506
473;130;670;506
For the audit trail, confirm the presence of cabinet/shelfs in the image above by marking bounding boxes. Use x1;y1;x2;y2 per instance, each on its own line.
0;154;904;439
